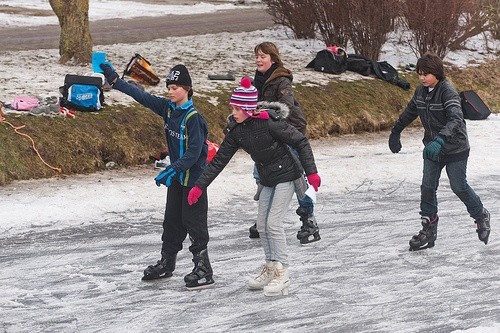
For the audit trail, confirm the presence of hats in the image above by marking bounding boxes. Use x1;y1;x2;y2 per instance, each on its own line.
166;64;192;88
229;77;269;120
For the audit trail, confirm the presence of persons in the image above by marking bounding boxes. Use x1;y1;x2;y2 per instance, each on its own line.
100;63;214;291
388;53;490;250
188;78;320;295
249;42;321;245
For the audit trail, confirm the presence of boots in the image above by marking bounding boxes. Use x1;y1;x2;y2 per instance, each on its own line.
295;204;323;247
184;248;216;291
248;223;261;241
140;248;177;278
408;214;439;253
472;205;492;247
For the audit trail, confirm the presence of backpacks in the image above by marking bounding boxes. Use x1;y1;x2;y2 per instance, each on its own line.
314;46;349;75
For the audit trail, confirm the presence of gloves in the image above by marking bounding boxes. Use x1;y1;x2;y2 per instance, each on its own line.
187;185;203;207
422;134;448;163
98;63;120;86
388;128;403;154
308;172;322;192
154;163;181;188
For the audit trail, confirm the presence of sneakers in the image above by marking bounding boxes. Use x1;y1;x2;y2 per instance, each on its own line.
262;262;302;301
246;260;277;292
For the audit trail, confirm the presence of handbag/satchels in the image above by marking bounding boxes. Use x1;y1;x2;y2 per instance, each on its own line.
460;88;490;121
120;53;161;86
59;73;106;113
